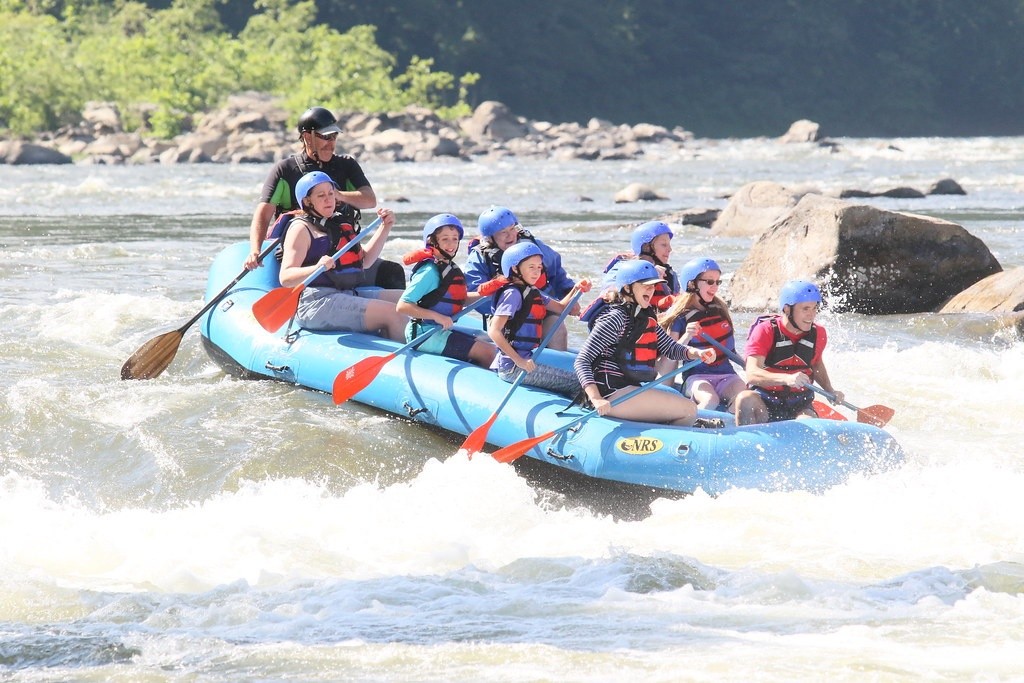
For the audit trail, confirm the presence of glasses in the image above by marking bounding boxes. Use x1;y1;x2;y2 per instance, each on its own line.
304;131;338;140
698;278;722;286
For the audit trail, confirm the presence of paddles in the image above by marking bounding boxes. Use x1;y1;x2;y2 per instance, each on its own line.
120;236;283;381
251;215;384;334
458;280;587;456
802;380;895;429
695;326;846;421
492;351;714;463
333;293;492;406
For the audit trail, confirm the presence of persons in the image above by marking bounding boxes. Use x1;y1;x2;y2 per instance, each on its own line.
278;171;409;346
465;207;580;350
244;106;376;271
489;241;593;398
632;221;681;386
396;212;497;369
736;280;844;427
573;260;716;426
659;258;746;414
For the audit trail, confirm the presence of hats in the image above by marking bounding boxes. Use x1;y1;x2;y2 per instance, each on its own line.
637;279;667;286
316;123;343;135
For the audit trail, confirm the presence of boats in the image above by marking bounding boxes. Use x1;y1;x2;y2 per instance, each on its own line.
195;237;904;498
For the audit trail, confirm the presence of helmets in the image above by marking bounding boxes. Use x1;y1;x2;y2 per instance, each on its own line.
615;259;658;293
681;258;721;293
781;281;822;313
422;214;463;250
295;171;335;212
478;207;518;238
298;107;337;135
631;221;673;257
500;242;543;279
